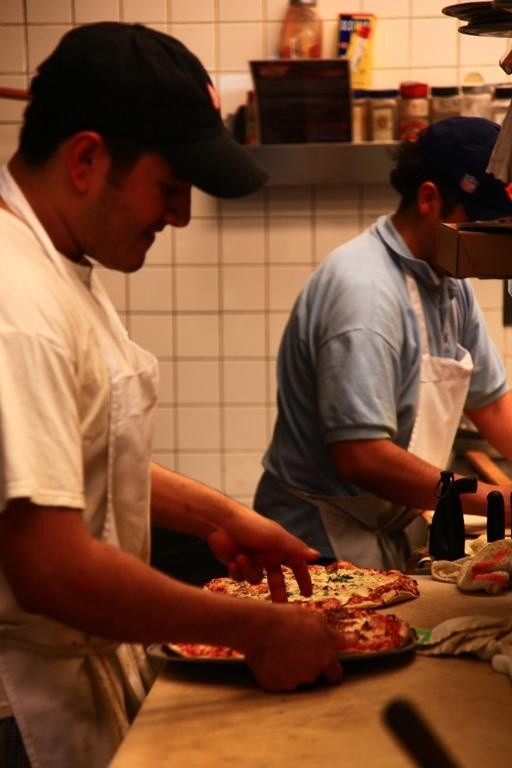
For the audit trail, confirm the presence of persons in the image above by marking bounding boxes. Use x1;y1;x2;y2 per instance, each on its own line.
252;113;512;577
2;18;348;768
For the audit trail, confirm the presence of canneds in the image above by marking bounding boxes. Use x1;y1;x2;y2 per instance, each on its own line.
350;83;511;141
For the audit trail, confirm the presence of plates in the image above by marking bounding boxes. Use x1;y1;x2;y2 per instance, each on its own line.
143;626;421;673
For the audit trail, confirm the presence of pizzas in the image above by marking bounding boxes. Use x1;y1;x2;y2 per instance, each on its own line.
203;561;420;609
167;598;417;660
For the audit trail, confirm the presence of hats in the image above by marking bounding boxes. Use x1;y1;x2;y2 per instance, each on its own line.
414;115;512;225
37;21;270;202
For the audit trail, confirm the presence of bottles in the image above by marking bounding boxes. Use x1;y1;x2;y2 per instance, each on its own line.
350;78;497;141
245;90;259;146
